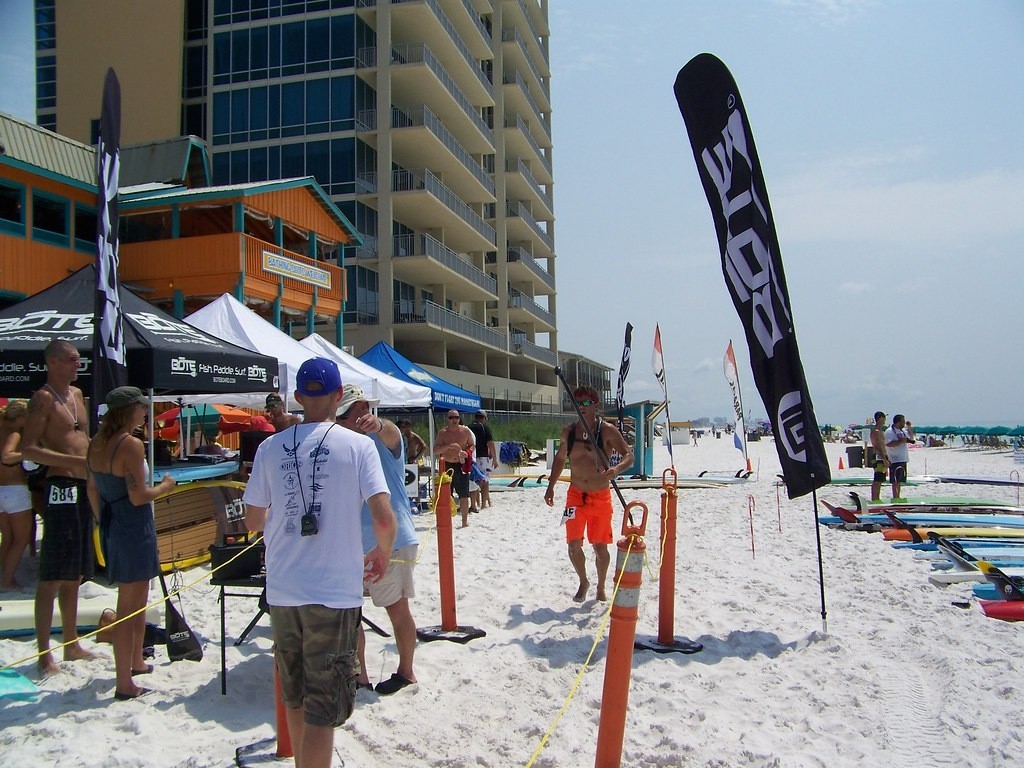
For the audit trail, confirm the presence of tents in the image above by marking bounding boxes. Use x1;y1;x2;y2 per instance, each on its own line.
0;264;484;590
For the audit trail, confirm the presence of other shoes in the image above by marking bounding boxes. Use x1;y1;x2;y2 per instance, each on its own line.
115;685;152;700
469;506;480;513
131;664;153;677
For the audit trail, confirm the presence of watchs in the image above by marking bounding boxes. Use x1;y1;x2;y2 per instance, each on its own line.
377;417;385;433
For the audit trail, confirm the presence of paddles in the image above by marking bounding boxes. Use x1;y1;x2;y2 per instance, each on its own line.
553;365;657;579
156;556;204;664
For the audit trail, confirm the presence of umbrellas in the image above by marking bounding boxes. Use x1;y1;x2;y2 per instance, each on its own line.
866;419;876;423
835;425;842;430
849;424;856;428
759;420;772;428
821;426;837;436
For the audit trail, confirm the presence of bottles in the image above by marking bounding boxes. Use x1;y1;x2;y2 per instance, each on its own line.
458;449;465;464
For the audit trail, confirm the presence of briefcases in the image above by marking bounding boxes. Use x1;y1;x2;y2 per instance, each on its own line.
207;532;265;580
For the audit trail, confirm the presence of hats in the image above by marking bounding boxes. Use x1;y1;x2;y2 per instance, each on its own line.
105;386;152;408
266;393;282;402
874;411;889;418
476;410;488;420
245;416;275;432
336;383;380;416
296;356;342;397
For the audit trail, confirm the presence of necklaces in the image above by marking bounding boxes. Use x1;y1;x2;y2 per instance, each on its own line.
583;431;588;440
45;383;78;427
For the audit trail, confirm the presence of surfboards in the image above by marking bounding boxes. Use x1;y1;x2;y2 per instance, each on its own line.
608;469;754;490
478;477;550;493
772;473;941;486
815;490;1024;622
909;474;1024;486
0;594;161;638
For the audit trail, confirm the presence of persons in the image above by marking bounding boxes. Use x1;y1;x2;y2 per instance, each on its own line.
0;391;497;594
21;339;110;675
544;386;634;602
885;415;913;498
871;411;891;503
86;386;174;700
693;423;1024;451
334;384;418;696
241;359;399;768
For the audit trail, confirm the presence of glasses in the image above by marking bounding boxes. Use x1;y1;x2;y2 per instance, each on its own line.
448;415;460;420
264;403;280;409
575;400;595;407
337;409;350;420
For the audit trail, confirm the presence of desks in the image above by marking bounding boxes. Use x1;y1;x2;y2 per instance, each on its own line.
210;577;267;698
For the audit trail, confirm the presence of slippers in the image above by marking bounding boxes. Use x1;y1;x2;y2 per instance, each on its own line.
375;673;418;694
